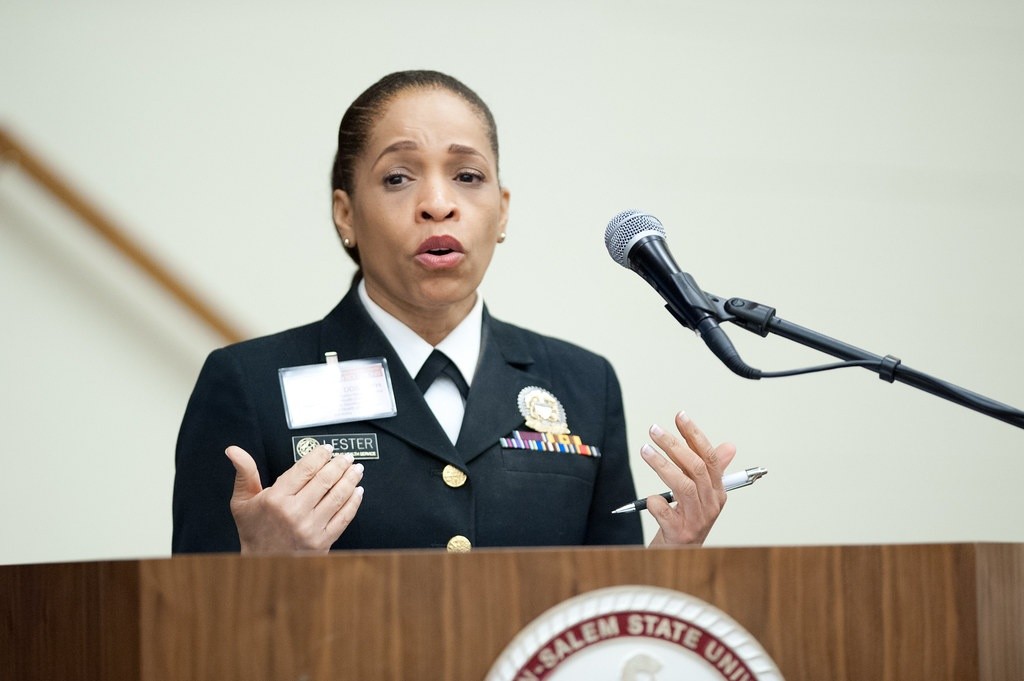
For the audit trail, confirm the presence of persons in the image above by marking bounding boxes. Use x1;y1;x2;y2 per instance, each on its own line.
170;70;736;557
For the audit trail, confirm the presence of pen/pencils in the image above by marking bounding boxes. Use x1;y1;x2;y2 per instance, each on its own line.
611;467;768;514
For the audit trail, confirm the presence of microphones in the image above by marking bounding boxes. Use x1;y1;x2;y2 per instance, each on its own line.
604;209;759;379
